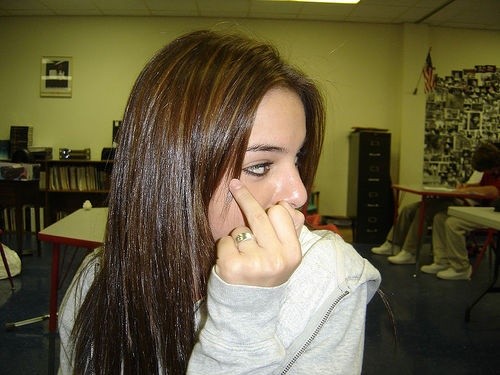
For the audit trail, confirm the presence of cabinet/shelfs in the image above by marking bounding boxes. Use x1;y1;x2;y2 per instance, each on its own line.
348;126;392;245
38;207;109;375
0;160;114;235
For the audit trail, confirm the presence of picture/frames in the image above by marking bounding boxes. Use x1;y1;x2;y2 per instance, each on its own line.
40;56;73;99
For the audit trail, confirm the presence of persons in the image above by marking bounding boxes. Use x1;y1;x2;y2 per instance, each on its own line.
371;142;500;281
56;21;399;375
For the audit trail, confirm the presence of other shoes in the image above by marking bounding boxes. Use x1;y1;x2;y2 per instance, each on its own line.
387;249;416;264
371;240;400;255
437;264;472;280
421;262;450;274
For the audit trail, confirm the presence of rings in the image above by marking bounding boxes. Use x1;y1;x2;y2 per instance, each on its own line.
234;232;255;244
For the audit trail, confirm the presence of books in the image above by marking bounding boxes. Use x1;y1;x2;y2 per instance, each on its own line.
0;160;41;181
7;125;53;160
59;147;90;160
1;204;76;235
39;165;112;192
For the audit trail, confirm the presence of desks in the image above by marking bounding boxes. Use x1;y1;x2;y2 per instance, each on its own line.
393;184;485;272
447;206;499;321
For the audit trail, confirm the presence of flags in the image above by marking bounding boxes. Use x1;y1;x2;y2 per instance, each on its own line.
423;52;435;92
